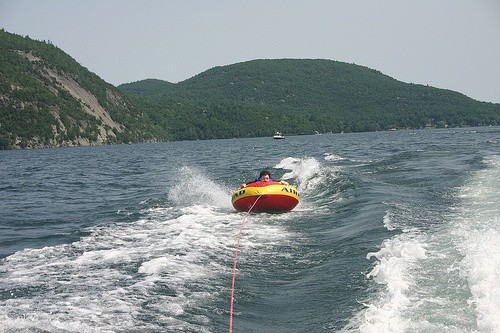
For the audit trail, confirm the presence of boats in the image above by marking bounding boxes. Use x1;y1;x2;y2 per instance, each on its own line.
273;132;285;139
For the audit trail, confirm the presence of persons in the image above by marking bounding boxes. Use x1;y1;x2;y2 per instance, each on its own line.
242;170;289;189
